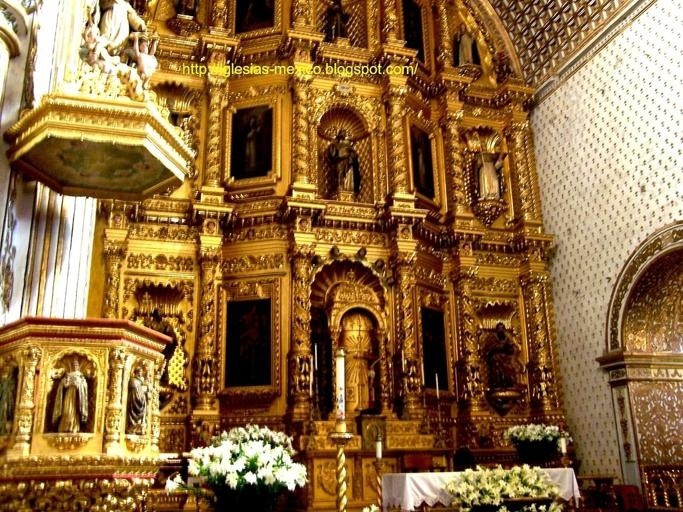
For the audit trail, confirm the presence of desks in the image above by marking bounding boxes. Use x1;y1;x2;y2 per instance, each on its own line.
383;469;581;512
577;475;618;507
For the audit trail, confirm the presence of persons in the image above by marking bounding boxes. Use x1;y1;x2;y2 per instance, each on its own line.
456;21;477;64
322;124;360;193
481;323;518;385
52;360;89;433
0;349;20;415
125;367;150;435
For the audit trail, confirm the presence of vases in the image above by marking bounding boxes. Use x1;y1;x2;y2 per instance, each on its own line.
472;498;552;512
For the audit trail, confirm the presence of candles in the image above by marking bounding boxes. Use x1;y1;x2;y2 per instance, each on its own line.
435;373;440;399
376;441;382;459
336;357;345;421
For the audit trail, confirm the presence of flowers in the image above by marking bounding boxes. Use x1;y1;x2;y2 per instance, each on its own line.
447;465;563;512
165;424;309;497
504;424;572;452
